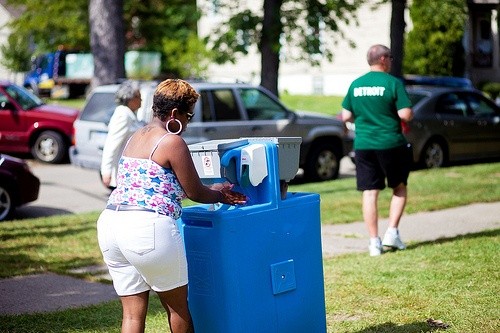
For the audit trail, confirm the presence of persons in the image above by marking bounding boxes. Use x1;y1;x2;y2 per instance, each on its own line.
96;79;247;333
100;79;146;191
342;44;415;257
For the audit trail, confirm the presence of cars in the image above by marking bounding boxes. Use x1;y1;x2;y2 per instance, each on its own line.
399;73;493;103
347;84;500;171
0;78;81;162
0;152;40;221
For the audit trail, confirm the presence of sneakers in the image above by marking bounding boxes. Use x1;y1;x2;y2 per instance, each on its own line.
381;228;406;250
369;237;382;257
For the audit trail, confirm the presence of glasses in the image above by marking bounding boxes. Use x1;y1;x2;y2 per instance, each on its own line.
178;107;195;121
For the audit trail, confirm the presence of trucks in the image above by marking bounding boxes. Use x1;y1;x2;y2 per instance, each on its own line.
22;44;162;101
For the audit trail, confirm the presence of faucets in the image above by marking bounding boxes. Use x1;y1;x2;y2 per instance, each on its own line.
220;146;240;168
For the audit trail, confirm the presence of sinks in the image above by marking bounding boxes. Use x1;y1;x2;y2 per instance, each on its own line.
177;203;272;217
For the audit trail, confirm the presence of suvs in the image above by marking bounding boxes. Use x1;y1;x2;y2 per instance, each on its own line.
69;77;355;192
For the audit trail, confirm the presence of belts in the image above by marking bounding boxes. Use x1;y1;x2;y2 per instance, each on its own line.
106;203;154;212
